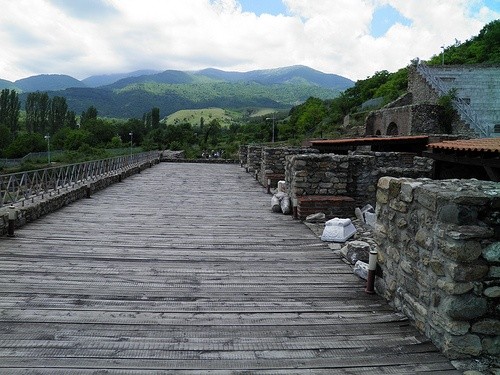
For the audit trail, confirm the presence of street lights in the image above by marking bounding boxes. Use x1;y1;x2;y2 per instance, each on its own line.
266;118;275;142
44;135;51;168
129;133;133;154
440;43;445;66
